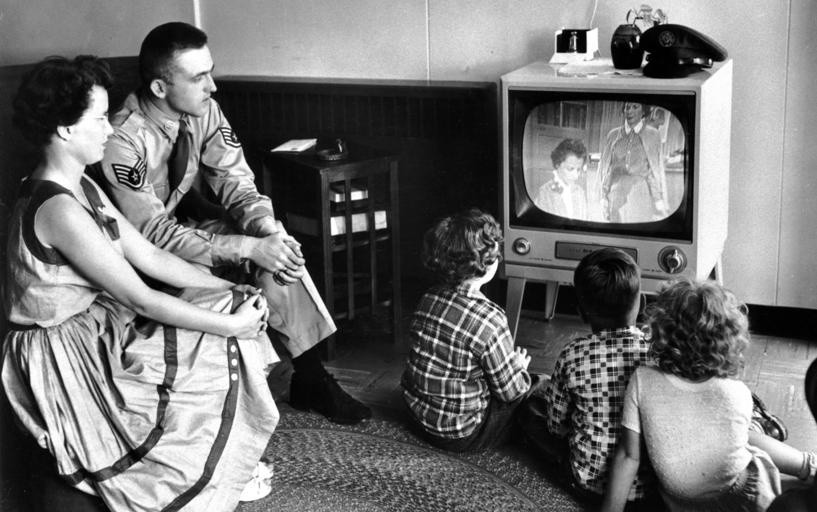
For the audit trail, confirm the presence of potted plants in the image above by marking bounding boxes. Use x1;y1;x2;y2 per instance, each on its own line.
610;4;667;69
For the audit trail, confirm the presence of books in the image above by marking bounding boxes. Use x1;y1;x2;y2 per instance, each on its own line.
329;185;368;203
269;137;317;154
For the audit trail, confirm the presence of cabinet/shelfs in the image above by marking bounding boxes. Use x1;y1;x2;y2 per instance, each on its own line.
267;153;401;362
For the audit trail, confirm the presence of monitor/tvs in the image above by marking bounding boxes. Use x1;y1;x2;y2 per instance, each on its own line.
499;56;735;282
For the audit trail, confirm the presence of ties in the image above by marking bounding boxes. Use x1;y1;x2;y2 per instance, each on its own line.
167;120;190;194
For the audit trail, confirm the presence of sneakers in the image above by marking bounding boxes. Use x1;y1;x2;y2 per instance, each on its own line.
751;392;788;442
287;369;372;427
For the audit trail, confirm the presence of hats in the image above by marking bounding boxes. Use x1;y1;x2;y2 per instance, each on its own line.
638;24;728;79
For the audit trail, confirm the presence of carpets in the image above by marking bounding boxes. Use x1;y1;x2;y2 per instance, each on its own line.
237;411;585;512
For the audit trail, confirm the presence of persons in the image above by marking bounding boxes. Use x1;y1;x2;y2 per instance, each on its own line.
0;53;282;512
534;139;591;220
86;21;372;426
516;244;659;512
761;361;817;511
599;276;816;512
401;209;551;454
599;102;670;223
